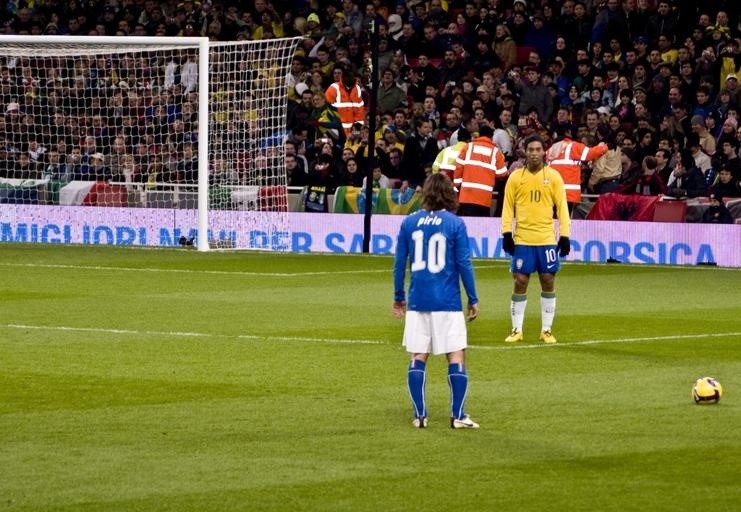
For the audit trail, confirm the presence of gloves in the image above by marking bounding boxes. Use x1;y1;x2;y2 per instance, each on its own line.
503;232;513;255
556;236;570;257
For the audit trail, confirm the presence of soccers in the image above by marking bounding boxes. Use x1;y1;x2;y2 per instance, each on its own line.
692;377;723;405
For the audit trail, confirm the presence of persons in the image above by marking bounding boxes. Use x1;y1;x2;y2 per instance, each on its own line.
1;0;739;224
393;174;480;429
502;137;572;344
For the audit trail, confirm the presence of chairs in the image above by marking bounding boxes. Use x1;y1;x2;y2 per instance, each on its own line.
404;44;532;100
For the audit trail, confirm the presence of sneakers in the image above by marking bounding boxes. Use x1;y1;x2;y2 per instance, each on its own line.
451;414;479;428
412;416;427;427
505;331;523;342
539;331;556;343
